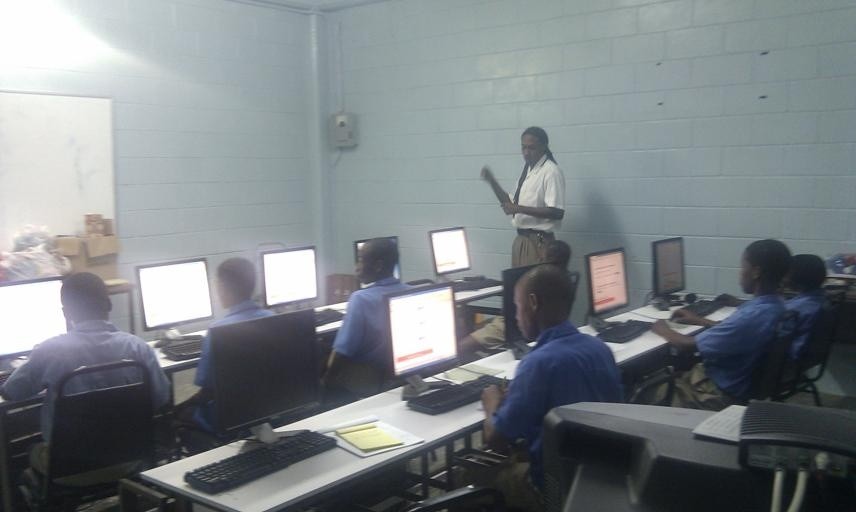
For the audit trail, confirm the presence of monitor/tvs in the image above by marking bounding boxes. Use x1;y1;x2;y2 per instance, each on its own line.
261;246;321;314
383;281;462;401
0;276;70;374
430;226;471;282
136;257;215;348
584;247;630;333
355;236;400;290
503;260;555;360
208;308;322;451
652;237;684;311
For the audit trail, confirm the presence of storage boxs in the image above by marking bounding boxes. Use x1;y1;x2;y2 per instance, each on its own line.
50;232;119;282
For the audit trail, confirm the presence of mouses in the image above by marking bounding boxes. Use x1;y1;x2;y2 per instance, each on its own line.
671;315;697;324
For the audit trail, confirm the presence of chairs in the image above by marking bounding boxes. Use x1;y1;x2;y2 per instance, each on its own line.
0;274;855;511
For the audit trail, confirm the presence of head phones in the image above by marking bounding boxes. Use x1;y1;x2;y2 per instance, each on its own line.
671;294;697;304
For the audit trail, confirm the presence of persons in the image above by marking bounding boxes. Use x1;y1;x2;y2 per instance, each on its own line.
633;239;790;415
446;264;626;510
670;253;831;380
176;256;282;427
1;269;174;489
320;238;419;393
478;126;566;267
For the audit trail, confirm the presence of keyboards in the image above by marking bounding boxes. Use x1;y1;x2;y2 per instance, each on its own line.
0;374;18;396
184;432;337;495
162;338;207;361
313;309;345;327
453;279;503;292
681;300;723;317
596;320;654;344
406;374;513;415
